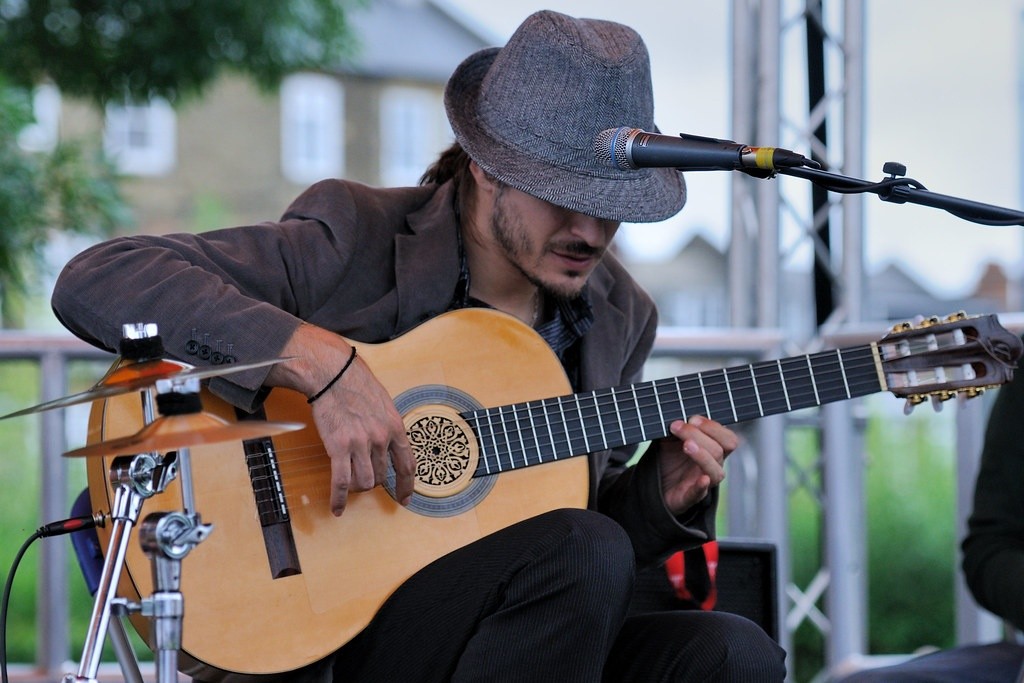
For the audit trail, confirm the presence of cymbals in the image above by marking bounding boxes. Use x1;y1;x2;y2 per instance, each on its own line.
0;353;298;421
59;410;308;459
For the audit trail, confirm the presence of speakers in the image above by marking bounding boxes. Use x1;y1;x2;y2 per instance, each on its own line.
717;541;779;644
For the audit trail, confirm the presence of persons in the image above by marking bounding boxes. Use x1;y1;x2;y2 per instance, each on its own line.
52;10;789;683
962;369;1024;637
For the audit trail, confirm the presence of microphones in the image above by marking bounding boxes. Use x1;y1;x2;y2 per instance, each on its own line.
593;126;806;171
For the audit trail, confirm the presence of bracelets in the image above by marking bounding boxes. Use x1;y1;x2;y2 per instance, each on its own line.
306;347;357;404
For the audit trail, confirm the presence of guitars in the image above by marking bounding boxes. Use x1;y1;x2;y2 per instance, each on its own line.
83;303;1024;683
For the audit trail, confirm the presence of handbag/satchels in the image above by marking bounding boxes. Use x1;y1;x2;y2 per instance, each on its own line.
625;546;777;645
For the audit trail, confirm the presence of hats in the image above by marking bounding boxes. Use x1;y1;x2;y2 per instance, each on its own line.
443;10;687;222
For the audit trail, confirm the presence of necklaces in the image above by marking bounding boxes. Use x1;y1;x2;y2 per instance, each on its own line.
532;286;541;327
